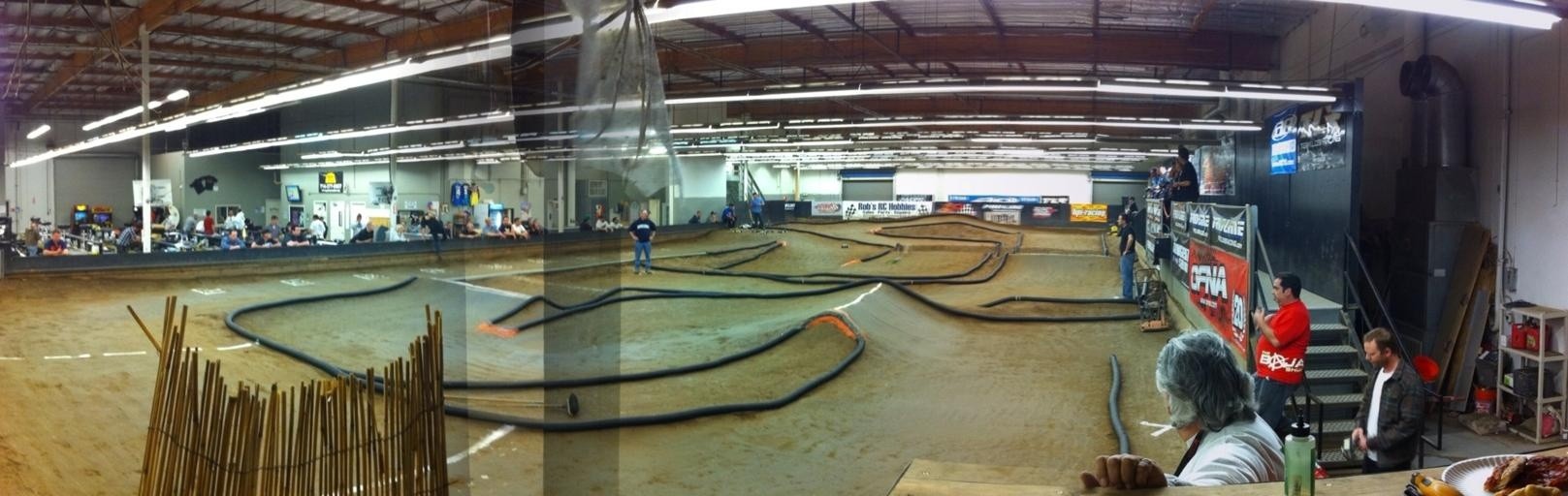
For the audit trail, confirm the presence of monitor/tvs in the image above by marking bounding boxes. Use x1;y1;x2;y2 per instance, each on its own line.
285;184;301;202
74;211;87;221
94;213;111;224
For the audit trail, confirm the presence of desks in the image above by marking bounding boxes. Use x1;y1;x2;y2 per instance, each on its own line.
886;449;1567;496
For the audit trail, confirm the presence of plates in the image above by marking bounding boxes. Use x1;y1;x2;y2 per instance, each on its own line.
1442;453;1535;495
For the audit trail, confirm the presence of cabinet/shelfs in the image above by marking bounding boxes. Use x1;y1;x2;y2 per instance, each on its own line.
1496;304;1567;445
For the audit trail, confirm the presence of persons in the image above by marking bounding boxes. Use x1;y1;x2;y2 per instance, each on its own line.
1080;329;1285;490
24;208;549;255
1352;327;1425;476
688;211;701;224
748;193;765;228
627;208;657;274
1125;147;1199;216
1251;271;1311;429
706;211;718;224
580;217;623;232
722;203;737;228
1115;213;1135;300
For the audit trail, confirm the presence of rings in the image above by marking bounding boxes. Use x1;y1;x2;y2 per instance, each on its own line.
1141;456;1150;463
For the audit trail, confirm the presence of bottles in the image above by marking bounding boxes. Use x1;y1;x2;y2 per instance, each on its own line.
1283;407;1315;495
